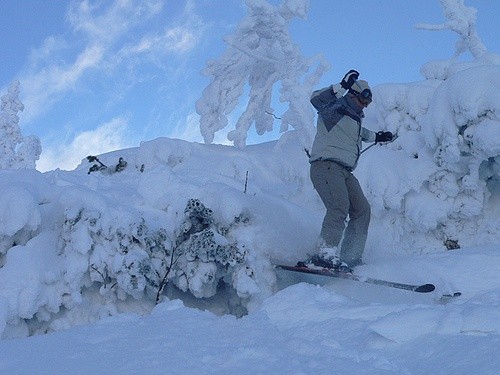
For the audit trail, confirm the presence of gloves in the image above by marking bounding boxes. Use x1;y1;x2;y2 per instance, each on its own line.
340;69;359;90
375;131;392;142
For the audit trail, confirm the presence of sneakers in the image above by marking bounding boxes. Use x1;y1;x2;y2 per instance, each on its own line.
316;252;353;272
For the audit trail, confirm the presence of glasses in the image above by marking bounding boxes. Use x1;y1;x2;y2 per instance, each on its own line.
358;95;371;107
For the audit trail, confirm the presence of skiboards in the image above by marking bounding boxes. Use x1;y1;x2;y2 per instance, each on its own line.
274;264;462;297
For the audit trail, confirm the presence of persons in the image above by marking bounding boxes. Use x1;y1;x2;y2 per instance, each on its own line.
88;156;127;175
309;70;392;273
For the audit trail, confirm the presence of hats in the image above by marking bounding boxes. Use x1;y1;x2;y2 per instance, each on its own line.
348;80;372;97
